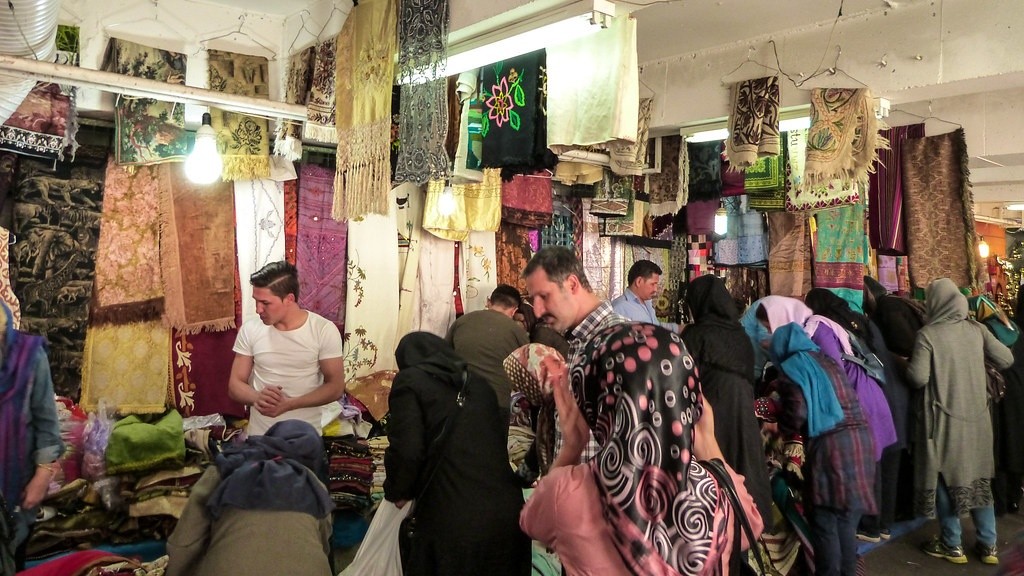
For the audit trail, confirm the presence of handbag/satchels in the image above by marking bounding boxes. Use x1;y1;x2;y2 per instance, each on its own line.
848;331;886;388
338;498;412;576
984;358;1005;403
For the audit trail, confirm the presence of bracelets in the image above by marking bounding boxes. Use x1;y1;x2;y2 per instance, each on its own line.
38;463;56;475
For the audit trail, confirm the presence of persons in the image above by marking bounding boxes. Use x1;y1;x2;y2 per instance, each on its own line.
907;277;1014;564
754;322;881;576
444;246;1024;543
0;297;66;576
520;321;763;576
167;420;337;576
228;261;344;441
383;331;532;576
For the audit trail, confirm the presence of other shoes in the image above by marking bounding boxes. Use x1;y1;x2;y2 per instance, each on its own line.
856;528;891;543
1008;501;1020;514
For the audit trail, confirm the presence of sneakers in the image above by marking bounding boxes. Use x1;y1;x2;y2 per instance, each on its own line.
975;540;998;564
923;538;968;563
